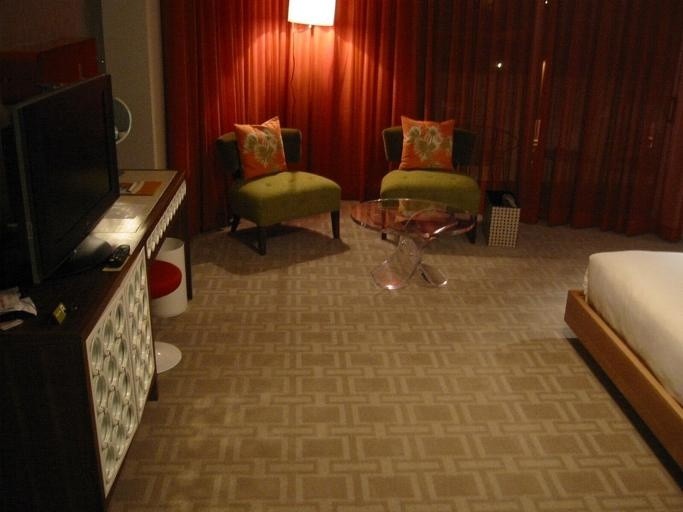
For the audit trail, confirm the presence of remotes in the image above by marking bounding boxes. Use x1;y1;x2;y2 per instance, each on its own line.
104;243;131;267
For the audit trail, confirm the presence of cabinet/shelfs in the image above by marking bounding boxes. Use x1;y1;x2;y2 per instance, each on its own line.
1;167;194;511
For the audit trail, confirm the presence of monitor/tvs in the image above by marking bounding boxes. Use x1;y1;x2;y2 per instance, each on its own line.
0;72;122;287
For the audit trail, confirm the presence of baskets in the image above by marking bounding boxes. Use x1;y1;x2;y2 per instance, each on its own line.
486;190;521;246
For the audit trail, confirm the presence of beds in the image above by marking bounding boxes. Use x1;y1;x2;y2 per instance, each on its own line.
565;250;683;479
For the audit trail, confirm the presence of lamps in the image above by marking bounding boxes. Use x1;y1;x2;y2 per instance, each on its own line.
287;1;335;28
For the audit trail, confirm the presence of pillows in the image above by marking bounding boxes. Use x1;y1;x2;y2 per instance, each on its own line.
396;115;459;172
233;115;288;182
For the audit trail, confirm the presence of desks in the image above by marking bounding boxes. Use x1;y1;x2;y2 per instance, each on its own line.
345;196;479;290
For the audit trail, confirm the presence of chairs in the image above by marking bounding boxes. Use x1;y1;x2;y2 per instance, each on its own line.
209;123;483;254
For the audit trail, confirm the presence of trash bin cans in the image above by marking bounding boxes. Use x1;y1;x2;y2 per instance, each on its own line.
148;237;187;319
